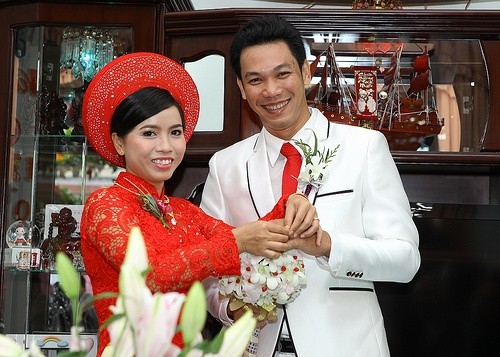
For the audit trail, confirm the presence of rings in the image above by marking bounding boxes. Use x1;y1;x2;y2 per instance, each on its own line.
314;218;319;220
284;225;290;231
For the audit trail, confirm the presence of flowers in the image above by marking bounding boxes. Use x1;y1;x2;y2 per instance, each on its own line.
288;128;340;194
139;191;177;229
217;252;307;323
55;226;256;357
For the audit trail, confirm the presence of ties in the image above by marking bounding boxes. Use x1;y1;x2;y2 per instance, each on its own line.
257;143;302;222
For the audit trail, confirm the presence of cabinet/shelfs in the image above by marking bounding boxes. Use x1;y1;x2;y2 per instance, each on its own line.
0;0;194;357
158;8;500;357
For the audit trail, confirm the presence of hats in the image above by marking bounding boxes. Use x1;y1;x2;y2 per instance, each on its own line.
81;51;200;168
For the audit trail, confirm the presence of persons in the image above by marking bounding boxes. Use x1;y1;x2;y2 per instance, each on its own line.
14;223;27;246
80;53;323;357
199;20;421;357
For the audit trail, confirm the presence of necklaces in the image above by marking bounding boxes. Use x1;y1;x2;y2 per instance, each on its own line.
114;181;170;204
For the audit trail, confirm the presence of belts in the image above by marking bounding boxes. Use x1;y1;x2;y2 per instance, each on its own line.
276;339;295;353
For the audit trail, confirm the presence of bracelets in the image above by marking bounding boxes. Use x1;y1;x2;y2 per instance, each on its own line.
286;193;309;207
